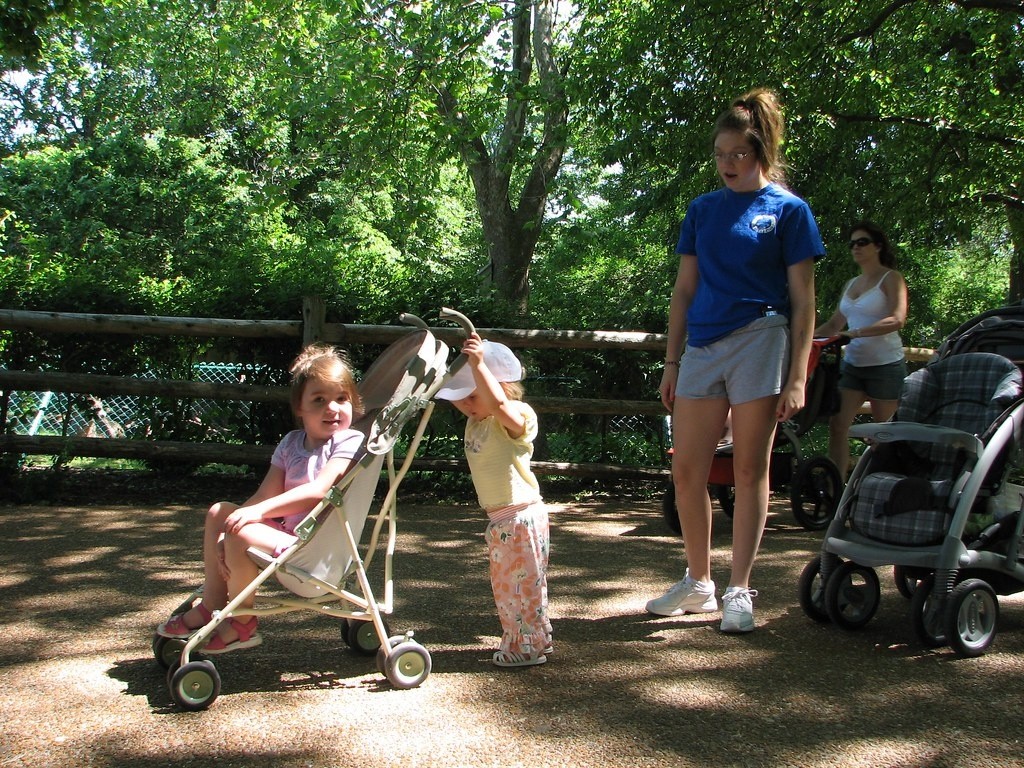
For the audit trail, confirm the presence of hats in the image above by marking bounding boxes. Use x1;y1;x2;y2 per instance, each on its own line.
434;339;521;401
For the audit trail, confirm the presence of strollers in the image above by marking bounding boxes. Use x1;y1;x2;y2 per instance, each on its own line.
151;306;478;712
797;295;1024;659
663;334;852;537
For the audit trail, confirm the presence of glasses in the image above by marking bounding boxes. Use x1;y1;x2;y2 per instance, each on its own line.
710;149;755;163
849;237;874;248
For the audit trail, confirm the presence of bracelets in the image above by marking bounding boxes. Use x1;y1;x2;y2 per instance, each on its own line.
664;361;679;366
854;328;861;337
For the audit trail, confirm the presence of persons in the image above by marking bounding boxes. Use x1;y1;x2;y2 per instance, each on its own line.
814;218;908;499
433;338;553;667
155;347;367;655
645;88;826;635
0;388;230;445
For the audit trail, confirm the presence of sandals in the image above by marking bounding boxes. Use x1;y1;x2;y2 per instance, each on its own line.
493;634;553;666
200;616;262;653
156;603;212;638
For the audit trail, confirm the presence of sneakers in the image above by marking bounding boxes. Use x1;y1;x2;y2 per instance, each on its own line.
720;586;759;631
644;567;717;616
